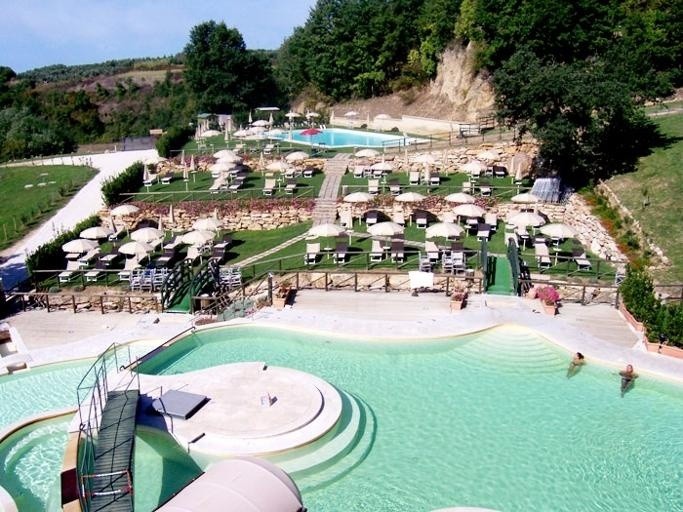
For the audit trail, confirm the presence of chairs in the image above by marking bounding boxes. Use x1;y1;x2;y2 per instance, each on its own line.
138;162;320;195
312;208;592;275
60;208;244;292
348;155;525;199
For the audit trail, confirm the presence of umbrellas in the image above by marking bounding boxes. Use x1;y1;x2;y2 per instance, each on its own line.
451;204;486;219
61;203;223;257
189;155;195;171
142;161;149;181
208;150;308;172
179;150;184;165
424;222;465;237
539;222;579;238
354;148;379;158
343;111;360;116
343;191;374;226
376;113;391;121
309;223;344;249
199;109;322;144
510;193;540;204
443;192;477;204
415;149;522;182
182;162;188;179
366;221;405;254
370;163;392;172
508;214;547;227
393;192;427;203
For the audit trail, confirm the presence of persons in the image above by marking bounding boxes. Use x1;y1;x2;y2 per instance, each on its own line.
618;362;638;399
565;352;584;380
